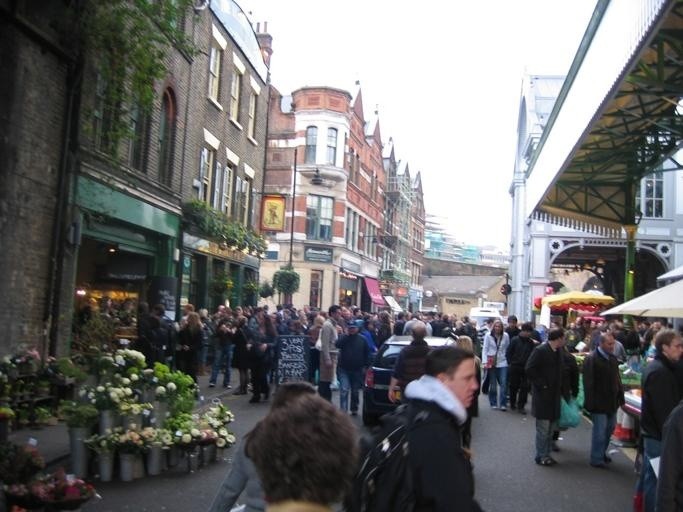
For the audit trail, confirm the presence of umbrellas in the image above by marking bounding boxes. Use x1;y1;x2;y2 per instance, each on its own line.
540;291;614;307
655;263;682;280
598;280;682;320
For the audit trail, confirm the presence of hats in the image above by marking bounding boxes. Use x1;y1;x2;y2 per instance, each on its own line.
547;326;566;341
346;321;359;328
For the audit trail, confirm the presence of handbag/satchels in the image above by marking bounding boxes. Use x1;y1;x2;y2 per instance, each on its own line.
486;354;498;368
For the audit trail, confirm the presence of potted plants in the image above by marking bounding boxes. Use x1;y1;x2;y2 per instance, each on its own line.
242;281;257;295
257;280;274;299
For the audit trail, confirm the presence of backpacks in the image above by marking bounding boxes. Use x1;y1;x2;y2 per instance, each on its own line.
343;403;457;512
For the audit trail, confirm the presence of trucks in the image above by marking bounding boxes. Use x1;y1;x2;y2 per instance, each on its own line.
470;307;500;327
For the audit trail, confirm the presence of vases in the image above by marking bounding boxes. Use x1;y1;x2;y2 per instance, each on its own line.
215;284;226;294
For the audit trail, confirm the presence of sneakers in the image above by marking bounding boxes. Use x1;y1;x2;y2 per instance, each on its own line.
487;402;529;414
207;379;272;403
535;455;553;466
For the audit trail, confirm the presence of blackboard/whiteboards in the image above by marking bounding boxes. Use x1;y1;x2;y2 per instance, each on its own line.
276;335;309;383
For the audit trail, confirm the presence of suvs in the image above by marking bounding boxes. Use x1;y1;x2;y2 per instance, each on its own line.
363;336;482;428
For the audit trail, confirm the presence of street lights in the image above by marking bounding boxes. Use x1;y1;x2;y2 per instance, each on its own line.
287;165;324;274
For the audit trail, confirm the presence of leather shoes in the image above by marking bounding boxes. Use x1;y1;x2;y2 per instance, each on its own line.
591;455;612;468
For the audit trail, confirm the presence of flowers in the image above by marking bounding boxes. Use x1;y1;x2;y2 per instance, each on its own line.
212;270;233;290
1;342;236;512
